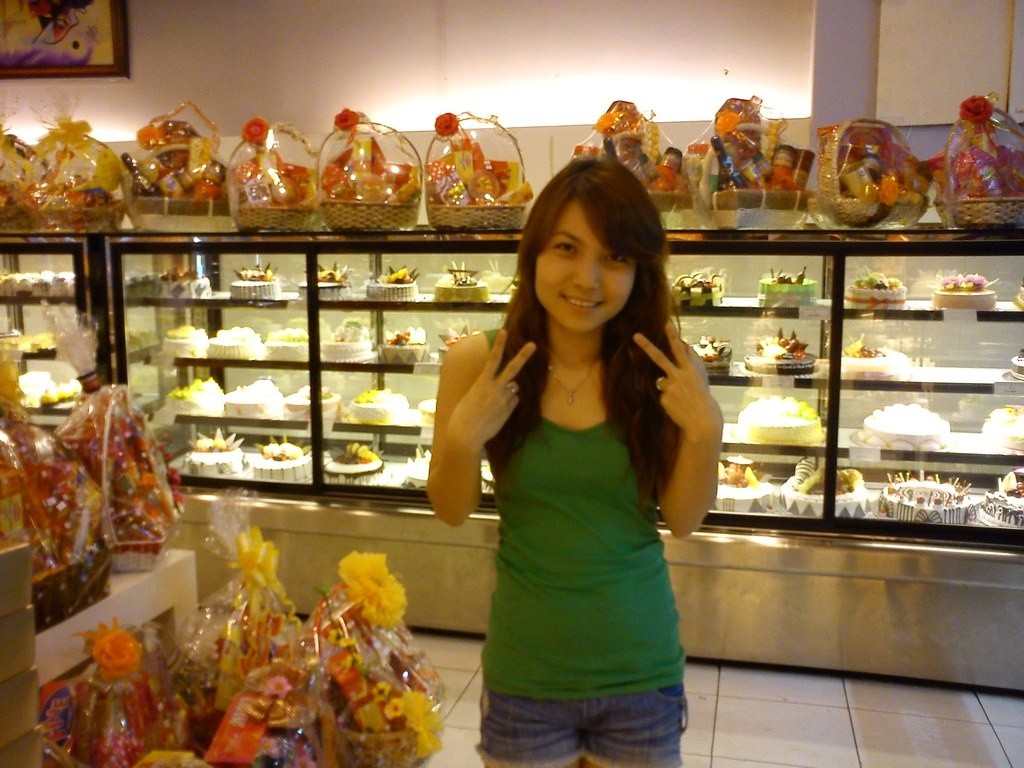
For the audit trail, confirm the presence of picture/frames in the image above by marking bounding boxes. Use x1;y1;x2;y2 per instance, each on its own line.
0;0;130;78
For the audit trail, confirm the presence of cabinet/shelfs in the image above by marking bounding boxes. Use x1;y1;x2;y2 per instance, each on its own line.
0;231;1024;693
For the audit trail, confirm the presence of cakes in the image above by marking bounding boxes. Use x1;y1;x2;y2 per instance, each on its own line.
670;266;1024;530
0;260;498;496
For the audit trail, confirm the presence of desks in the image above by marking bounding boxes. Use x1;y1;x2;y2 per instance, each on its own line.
35;546;199;691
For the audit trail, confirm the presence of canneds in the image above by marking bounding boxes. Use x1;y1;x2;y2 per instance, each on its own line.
738;159;766;187
773;144;796;170
154;172;184;197
659;147;683;173
861;152;881;182
204;159;227;186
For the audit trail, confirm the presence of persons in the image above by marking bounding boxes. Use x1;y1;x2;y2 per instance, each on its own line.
425;155;722;768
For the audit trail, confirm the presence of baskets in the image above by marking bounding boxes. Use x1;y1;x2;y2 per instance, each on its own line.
179;601;274;751
0;134;50;233
423;117;528;232
128;143;234;234
0;421;113;630
313;588;445;768
318;121;423;231
94;385;167;571
807;118;929;229
22;133;127;233
933;107;1023;226
691;123;814;229
605;108;691;231
225;126;324;232
60;619;178;768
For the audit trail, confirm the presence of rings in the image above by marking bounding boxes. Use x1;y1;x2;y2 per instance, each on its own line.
507;384;517;395
656;378;666;391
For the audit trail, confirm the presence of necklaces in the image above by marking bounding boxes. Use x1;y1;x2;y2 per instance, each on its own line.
549;357;598;405
